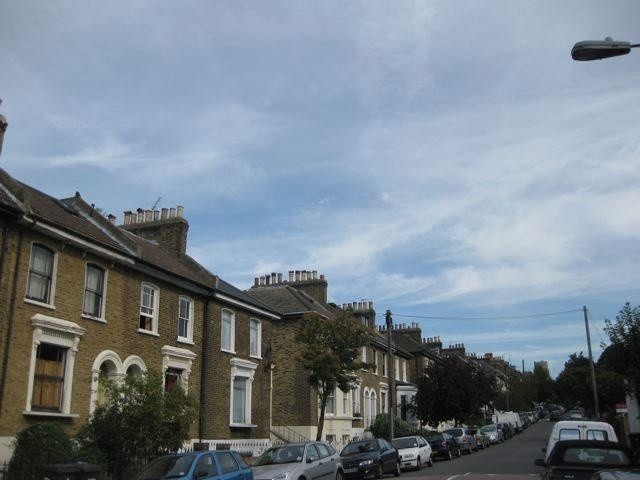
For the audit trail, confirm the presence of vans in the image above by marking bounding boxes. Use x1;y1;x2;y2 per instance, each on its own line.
539;418;621;463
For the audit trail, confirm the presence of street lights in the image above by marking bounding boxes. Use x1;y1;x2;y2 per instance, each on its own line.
570;36;639;62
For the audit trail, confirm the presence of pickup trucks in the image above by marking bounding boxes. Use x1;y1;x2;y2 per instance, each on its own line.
533;437;640;480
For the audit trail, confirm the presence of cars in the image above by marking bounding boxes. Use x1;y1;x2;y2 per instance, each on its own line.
131;448;256;480
423;431;461;461
249;439;346;480
339;436;402;480
446;400;585;455
390;434;435;472
